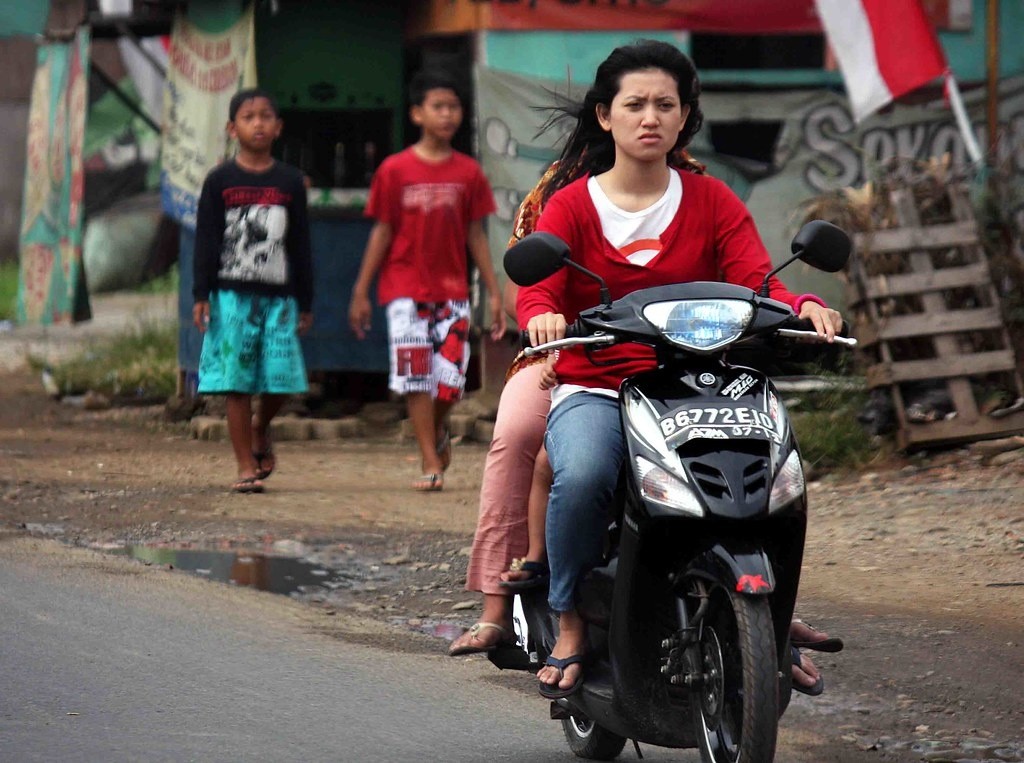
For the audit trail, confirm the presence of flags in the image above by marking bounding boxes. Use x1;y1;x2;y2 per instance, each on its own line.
816;0;948;124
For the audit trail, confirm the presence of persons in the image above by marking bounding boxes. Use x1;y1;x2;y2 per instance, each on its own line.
192;88;316;491
449;38;843;700
350;83;507;492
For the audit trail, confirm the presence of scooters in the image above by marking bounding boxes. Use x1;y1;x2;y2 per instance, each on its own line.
488;218;862;763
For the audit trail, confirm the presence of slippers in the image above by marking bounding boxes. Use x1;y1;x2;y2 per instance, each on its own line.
539;655;588;698
449;623;517;656
438;424;451;471
252;447;273;479
792;645;824;696
419;474;443;492
237;477;263;493
791;618;844;653
